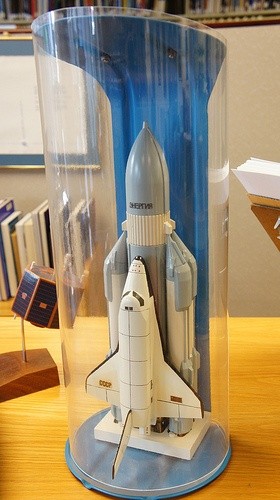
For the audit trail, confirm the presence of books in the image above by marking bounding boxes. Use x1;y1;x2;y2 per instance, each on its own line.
230;156;280;254
1;196;99;302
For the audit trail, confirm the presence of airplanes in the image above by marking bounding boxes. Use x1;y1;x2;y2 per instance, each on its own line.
84;120;204;480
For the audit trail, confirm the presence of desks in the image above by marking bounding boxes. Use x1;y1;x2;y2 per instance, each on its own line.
0;313;280;499
